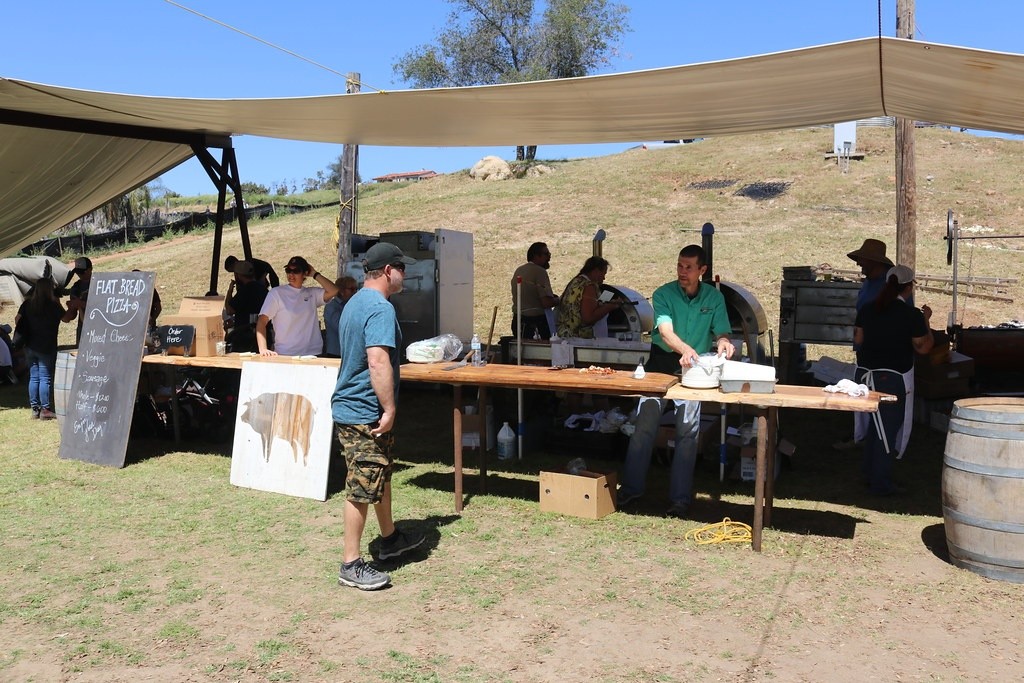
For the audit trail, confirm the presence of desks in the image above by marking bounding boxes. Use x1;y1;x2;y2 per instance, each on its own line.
142;350;898;552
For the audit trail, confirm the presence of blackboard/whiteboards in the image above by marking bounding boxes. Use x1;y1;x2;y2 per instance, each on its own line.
159;324;195;347
230;360;339;502
58;271;157;469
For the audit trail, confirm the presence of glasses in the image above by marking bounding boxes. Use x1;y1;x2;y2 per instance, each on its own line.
388;264;405;273
285;267;304;274
343;286;357;292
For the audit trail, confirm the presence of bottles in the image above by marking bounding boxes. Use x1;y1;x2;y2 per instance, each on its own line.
497;422;517;460
470;332;482;365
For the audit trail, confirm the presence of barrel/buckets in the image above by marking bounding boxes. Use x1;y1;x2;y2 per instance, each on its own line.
54;348;78;435
942;396;1024;584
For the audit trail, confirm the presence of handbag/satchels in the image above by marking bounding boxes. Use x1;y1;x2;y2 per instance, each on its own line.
13;314;30;348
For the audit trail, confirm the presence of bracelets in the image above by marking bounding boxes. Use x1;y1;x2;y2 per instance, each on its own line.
313;272;319;279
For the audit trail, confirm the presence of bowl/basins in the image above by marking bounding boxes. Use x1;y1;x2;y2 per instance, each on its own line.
681;364;721;387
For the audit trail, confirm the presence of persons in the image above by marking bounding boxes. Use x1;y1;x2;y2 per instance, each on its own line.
224;255;280;353
554;257;624;340
14;278;76;421
131;269;161;354
256;256;338;357
67;256;92;347
323;276;358;358
511;239;559;341
848;238;934;510
331;241;425;590
611;246;735;517
0;327;13;403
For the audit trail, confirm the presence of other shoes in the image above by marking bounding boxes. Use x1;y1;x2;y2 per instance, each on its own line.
666;503;688;519
884;481;906;495
832;438;865;451
32;410;40;419
622;498;650;516
39;408;57;420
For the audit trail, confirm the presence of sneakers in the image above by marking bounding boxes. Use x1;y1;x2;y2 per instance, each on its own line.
338;558;391;590
379;532;425;560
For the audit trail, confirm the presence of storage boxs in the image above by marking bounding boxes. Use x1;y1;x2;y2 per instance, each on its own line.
461;403;495;451
656;410;719;452
540;462;617;520
161;295;227;357
728;436;796;480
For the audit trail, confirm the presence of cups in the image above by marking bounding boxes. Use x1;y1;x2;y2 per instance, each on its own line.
464;406;473;414
215;341;226;357
824;269;832;282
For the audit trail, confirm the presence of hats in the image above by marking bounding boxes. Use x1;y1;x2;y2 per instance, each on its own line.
283;256;309;270
847;239;895;267
886;265;917;285
71;257;92;275
362;241;417;272
